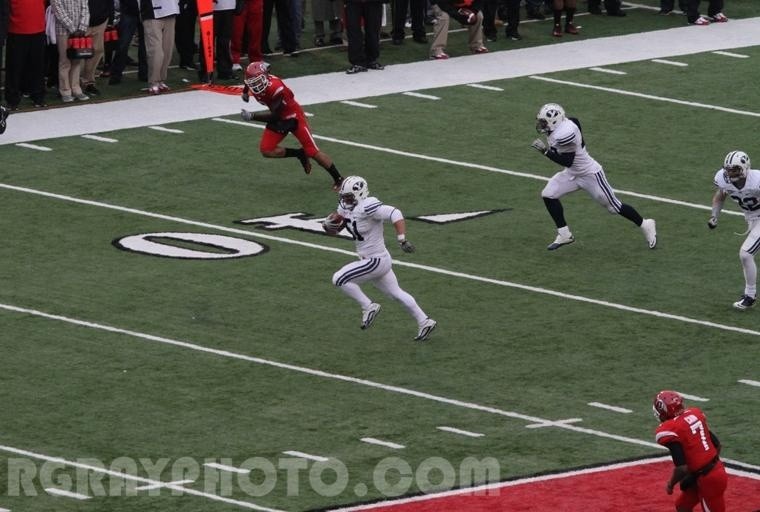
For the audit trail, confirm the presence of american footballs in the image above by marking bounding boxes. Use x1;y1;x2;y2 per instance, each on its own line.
329;215;346;232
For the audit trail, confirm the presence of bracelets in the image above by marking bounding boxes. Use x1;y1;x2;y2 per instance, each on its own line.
396;235;405;241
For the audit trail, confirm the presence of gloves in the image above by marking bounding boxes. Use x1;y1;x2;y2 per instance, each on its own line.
242;107;251;120
397;233;416;254
708;215;718;227
241;92;250;100
323;213;338;232
531;138;548;154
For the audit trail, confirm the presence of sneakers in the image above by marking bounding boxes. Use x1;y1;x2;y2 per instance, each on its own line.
61;92;90;103
361;302;381;329
547;233;574;249
713;12;728;22
552;22;562;36
565;23;580;34
371;60;383;70
414;318;436;342
695;17;708;25
298;149;314;174
232;63;242;71
148;83;169;94
346;64;369;75
332;178;345;189
640;218;657;248
733;294;757;308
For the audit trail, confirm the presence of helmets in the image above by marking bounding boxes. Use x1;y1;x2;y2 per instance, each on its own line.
653;390;685;423
723;150;750;182
337;176;369;210
245;61;270;93
536;103;566;134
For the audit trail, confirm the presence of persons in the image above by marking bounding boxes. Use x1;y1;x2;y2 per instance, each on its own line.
240;60;343;193
526;103;659;255
648;389;732;512
0;1;729;116
704;150;759;312
320;176;437;344
